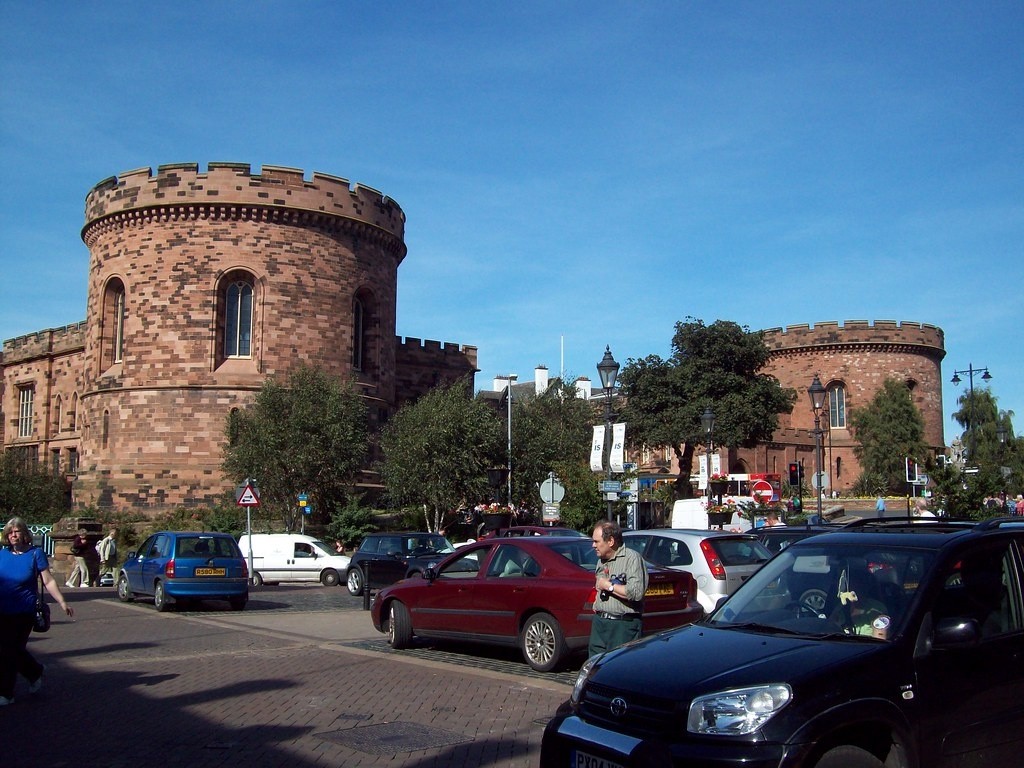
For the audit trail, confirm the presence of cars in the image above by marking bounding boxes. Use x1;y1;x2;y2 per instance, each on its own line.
491;523;845;616
117;531;250;612
370;535;705;672
535;517;1024;768
346;532;479;596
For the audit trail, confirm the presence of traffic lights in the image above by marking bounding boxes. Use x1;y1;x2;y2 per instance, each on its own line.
789;462;799;485
916;486;925;496
794;497;801;513
907;457;916;480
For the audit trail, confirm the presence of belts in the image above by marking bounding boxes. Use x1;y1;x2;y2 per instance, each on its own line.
596;610;641;621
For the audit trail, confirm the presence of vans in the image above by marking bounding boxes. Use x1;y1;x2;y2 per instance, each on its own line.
236;534;352;586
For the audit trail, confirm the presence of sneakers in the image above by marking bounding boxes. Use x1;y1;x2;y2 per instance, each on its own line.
65;582;75;588
0;695;15;706
28;662;48;696
80;584;89;588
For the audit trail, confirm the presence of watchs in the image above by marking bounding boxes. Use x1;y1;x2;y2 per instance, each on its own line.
608;583;615;593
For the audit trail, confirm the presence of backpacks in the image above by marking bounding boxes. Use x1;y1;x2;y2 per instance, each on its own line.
95;538;109;558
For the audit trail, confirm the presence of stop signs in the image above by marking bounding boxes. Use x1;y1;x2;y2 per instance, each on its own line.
752;481;773;505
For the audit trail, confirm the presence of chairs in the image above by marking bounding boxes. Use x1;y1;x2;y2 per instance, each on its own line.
935;550;1012;639
195;541;211;558
499;556;527;578
656;541;690;567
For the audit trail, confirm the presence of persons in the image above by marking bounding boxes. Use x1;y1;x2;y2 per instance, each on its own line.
588;521;649;659
335;540;344;556
0;517;73;706
876;495;884;517
65;528;90;587
916;503;938;523
92;528;120;587
765;512;788;551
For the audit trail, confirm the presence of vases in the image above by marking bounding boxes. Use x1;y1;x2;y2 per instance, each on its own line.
710;481;729;495
706;512;733;524
483;514;513;529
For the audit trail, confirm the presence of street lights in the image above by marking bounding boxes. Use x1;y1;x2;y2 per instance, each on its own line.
495;375;518;506
995;418;1008;513
950;363;993;458
596;345;621;520
807;372;827;517
701;404;717;509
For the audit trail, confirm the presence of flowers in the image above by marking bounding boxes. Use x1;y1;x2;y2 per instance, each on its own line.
699;499;743;518
475;502;511;513
707;468;729;482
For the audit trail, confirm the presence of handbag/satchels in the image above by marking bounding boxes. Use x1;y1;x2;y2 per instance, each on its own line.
69;545;81;554
33;603;50;633
